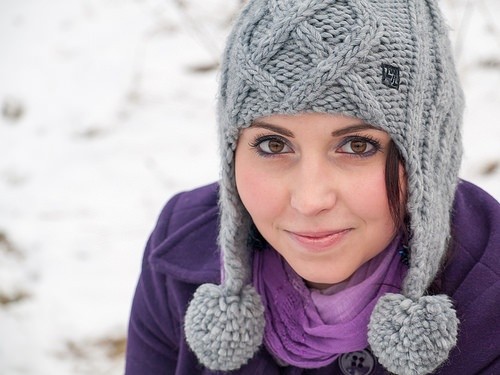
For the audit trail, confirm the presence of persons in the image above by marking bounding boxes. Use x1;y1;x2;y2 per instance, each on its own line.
126;1;499;375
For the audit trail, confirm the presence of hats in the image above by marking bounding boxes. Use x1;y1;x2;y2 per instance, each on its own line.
182;0;461;375
